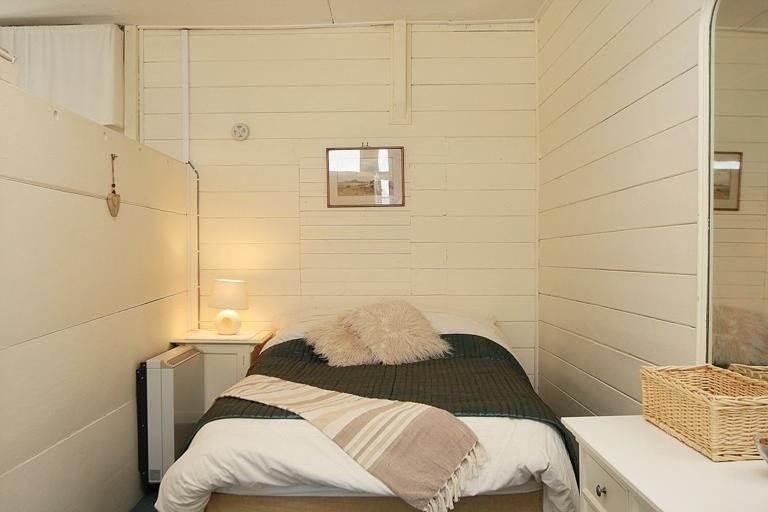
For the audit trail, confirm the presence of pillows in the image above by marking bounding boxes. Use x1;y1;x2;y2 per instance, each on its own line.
713;303;767;368
343;300;453;366
304;318;379;367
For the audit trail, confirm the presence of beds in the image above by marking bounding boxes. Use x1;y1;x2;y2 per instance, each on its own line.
154;310;579;512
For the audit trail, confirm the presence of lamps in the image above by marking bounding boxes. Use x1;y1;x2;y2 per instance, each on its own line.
208;279;248;335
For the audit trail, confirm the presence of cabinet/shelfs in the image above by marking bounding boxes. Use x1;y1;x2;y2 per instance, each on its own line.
560;415;768;511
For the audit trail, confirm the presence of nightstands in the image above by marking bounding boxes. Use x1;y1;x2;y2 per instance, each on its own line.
170;329;273;415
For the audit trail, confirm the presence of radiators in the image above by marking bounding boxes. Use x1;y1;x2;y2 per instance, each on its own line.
136;346;203;490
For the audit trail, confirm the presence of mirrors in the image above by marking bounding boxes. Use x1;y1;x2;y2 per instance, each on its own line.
706;1;768;388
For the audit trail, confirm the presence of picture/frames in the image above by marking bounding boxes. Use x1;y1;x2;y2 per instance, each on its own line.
326;146;405;208
713;151;743;211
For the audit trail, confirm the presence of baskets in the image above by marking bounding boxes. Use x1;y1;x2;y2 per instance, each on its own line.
727;364;767;383
641;362;767;462
